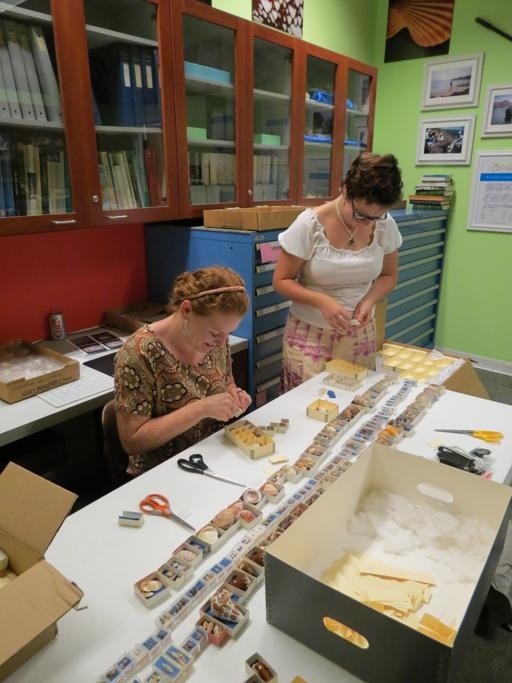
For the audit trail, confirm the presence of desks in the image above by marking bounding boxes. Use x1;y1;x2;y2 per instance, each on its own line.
0;368;511;682
0;302;248;449
185;204;451;412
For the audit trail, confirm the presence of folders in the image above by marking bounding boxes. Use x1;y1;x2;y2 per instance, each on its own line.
91;40;161;127
0;21;63;123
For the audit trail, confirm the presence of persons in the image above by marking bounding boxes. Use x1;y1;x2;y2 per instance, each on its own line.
271;151;404;398
108;263;254;482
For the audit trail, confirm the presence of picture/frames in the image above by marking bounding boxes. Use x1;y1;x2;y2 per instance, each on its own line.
419;52;484;110
480;85;512;138
466;150;512;235
415;112;477;166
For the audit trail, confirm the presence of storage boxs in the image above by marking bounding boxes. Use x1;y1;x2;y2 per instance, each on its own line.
0;461;84;683
262;441;512;683
368;300;492;399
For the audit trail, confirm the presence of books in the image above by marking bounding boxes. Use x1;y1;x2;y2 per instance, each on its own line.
408;172;454;211
0;128;163;218
189;152;292;206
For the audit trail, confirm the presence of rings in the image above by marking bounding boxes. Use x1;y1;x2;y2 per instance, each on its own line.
236;389;243;394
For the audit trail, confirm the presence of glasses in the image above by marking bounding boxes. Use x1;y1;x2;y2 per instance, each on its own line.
352;198;387;222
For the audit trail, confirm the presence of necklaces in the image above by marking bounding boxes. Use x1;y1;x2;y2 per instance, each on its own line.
335;195;358;245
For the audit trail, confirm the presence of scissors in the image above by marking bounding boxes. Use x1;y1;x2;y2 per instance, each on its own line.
434;429;504;443
140;494;197;532
178;453;246;487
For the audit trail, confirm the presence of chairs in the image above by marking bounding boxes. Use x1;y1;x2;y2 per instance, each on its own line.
101;398;129;487
